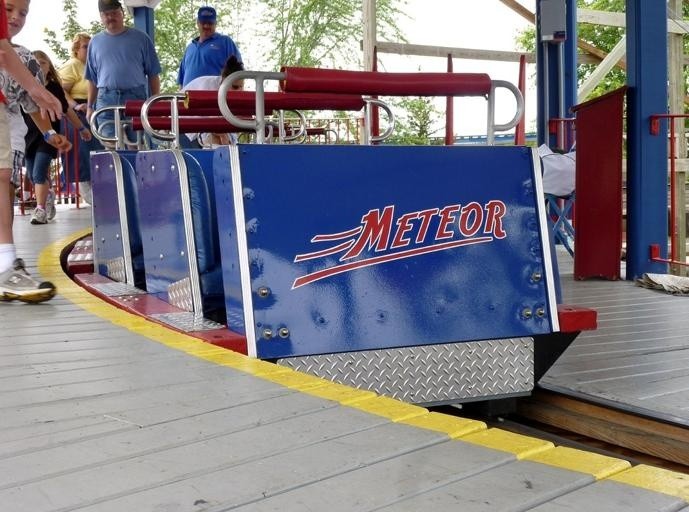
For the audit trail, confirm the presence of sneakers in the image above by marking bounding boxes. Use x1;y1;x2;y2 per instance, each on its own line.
0;257;57;305
18;197;37;210
30;207;48;224
45;188;57;220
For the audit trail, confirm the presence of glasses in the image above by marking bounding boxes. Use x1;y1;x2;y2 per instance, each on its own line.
231;84;241;91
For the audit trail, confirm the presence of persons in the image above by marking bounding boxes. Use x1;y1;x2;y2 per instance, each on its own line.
175;7;243;91
0;0;91;303
171;54;243;149
85;0;162;149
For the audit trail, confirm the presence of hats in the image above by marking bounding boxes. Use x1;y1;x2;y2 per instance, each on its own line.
98;0;121;12
197;7;216;24
